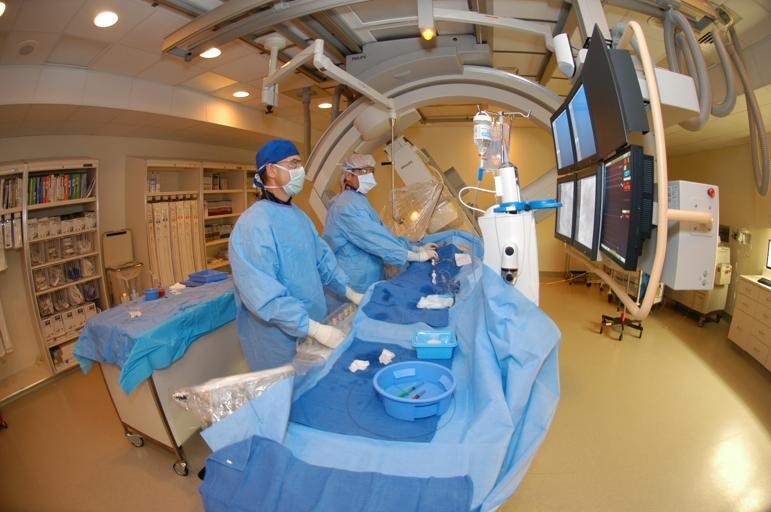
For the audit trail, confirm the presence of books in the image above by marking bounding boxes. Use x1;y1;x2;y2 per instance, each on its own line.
0;171;95;206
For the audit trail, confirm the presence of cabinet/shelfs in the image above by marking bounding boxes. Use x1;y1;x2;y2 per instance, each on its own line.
127;155;262;290
1;158;112;404
728;274;770;373
662;247;733;327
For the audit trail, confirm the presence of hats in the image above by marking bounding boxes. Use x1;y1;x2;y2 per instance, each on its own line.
255;139;300;176
342;154;376;169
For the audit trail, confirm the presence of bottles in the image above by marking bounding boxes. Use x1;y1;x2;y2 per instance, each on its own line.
473;120;493;157
121;293;130;310
131;288;140;304
150;171;161;192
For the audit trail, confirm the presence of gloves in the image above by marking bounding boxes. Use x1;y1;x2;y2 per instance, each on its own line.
345;286;365;305
307;318;346;349
408;242;440;262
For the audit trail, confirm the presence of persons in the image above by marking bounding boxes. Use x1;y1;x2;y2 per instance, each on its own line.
323;152;439;312
227;137;365;375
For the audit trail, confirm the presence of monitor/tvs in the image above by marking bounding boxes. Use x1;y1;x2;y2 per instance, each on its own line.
574;169;601;259
601;144;651;272
554;176;575;245
582;24;647;159
566;80;596;167
550;104;576;174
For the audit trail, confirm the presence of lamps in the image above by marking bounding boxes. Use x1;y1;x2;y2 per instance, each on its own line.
416;0;436;43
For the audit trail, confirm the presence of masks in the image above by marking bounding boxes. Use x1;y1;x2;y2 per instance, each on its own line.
275;164;305;197
355;172;377;194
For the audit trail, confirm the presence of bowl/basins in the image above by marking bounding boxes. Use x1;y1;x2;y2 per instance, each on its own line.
374;362;457;419
144;288;160;301
